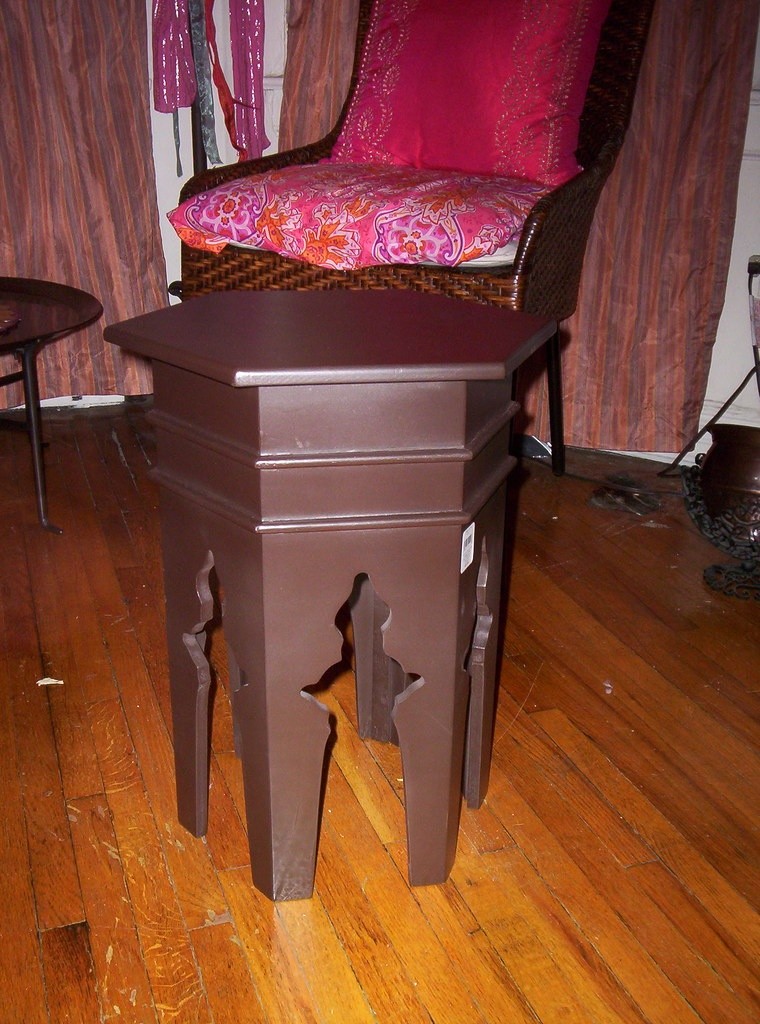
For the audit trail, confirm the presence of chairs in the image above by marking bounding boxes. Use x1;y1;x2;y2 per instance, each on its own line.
165;0;653;476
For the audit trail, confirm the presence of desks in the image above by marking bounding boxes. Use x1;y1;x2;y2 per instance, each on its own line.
102;287;561;901
0;277;102;533
